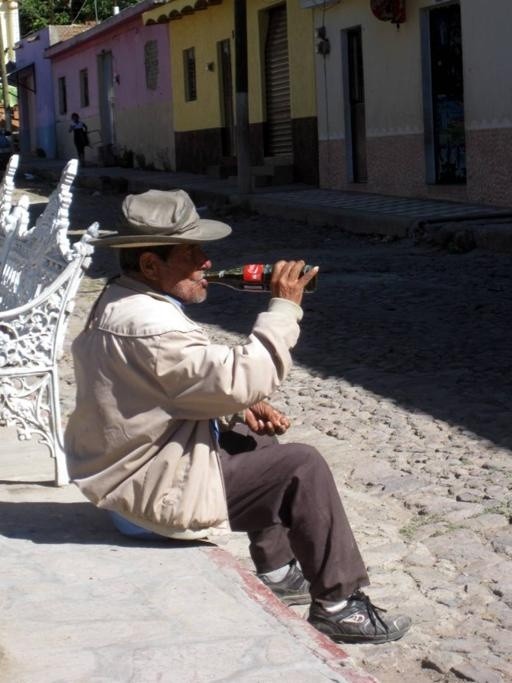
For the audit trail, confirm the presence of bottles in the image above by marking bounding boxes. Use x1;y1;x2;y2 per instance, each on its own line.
203;263;316;294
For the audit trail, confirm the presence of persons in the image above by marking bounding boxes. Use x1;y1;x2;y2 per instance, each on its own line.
61;186;415;647
0;119;13;156
67;112;89;162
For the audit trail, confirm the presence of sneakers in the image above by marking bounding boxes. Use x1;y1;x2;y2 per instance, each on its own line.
257;563;312;607
306;592;411;645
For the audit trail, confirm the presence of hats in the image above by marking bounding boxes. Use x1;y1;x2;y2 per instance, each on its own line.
87;188;233;250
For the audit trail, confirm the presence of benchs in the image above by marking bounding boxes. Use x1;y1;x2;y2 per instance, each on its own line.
0;153;102;486
